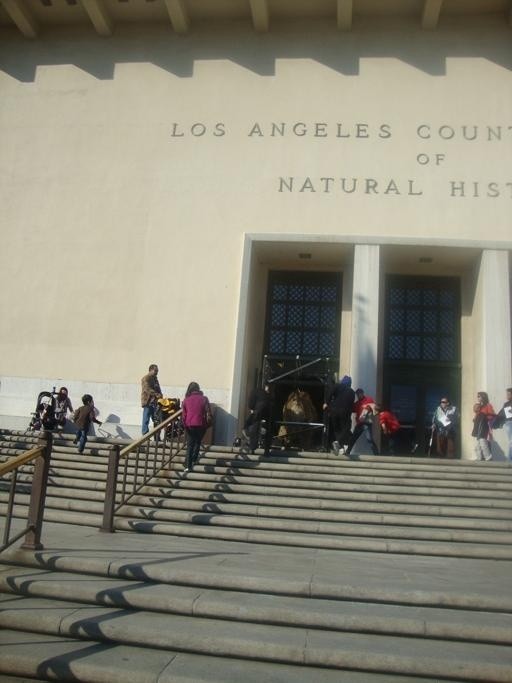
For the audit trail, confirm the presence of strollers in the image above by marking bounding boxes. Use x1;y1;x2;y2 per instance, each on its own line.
154;397;182;440
30;393;67;432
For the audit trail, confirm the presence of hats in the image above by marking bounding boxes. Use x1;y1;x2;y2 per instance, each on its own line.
342;374;350;386
355;389;366;394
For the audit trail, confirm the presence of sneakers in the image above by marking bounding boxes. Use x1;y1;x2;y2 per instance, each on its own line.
184;468;192;472
332;441;340;456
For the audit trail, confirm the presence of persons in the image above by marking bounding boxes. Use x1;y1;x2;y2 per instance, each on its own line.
345;387;379;455
430;396;458;457
72;393;102;454
52;386;75;433
322;375;355;453
375;402;401;455
493;387;511;429
142;362;164;442
469;391;492;460
248;386;271;457
182;381;212;473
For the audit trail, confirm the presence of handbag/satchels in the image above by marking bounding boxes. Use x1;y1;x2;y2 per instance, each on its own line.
205;402;215;427
